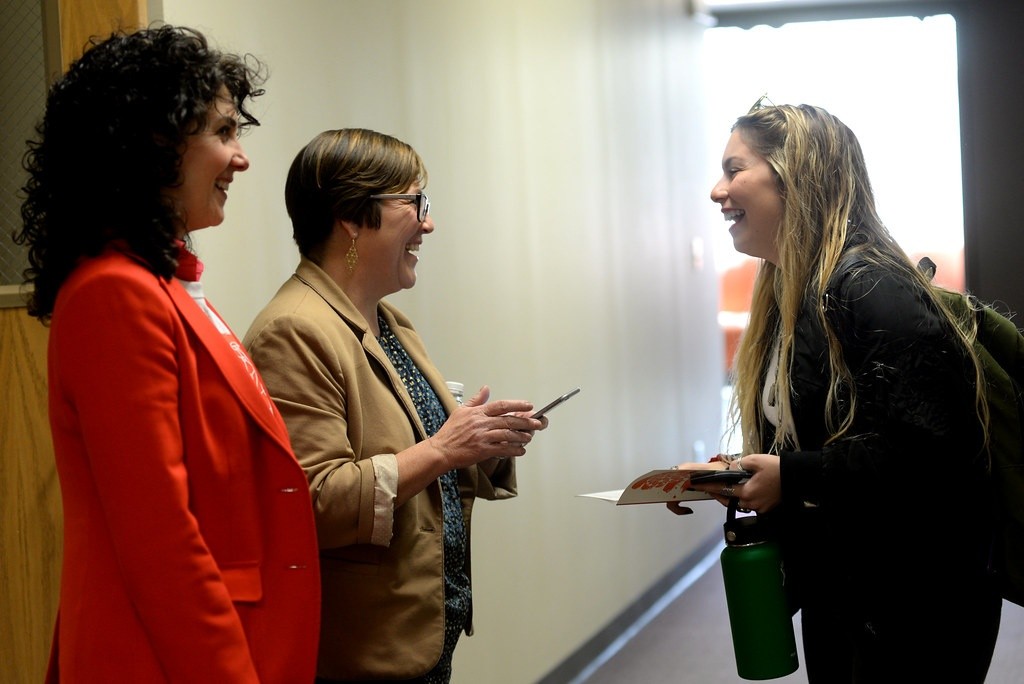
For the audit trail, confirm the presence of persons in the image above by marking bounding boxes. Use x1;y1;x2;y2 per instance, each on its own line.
666;91;1004;684
14;20;324;684
243;128;549;684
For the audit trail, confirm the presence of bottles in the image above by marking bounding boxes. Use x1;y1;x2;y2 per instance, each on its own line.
445;383;464;406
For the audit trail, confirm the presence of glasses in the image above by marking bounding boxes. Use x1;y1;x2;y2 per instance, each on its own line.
370;194;430;223
747;92;787;122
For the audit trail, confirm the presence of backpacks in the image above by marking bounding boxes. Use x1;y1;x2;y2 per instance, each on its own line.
830;243;1024;608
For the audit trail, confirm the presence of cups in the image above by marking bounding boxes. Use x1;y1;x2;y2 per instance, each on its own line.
720;499;799;680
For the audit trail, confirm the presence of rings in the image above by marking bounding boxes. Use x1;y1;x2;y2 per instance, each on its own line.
720;485;735;496
670;465;679;470
738;457;746;472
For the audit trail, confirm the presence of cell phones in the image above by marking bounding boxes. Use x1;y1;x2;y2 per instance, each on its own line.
517;388;581;432
